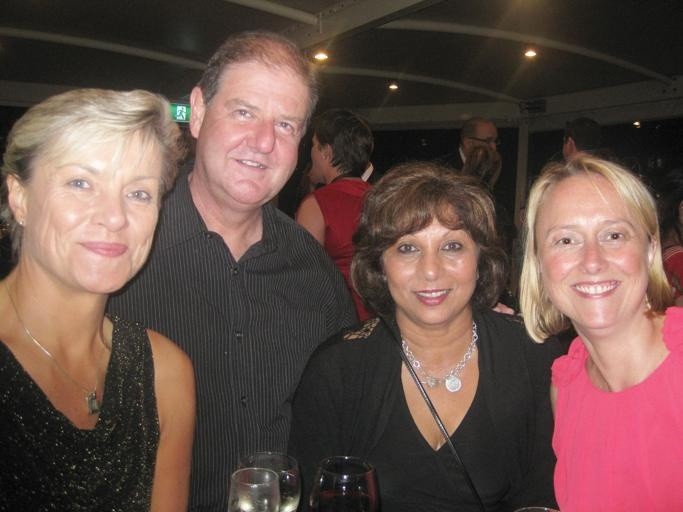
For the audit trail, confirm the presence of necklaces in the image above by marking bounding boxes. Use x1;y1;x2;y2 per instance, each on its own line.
401;320;480;393
3;278;104;415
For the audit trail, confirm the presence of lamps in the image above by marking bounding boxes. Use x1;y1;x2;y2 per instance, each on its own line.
524;45;537;58
315;47;328;61
388;80;399;89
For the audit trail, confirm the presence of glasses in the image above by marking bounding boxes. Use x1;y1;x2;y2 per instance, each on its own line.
468;137;501;144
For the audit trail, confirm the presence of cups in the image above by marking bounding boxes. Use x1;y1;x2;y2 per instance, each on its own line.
225;467;281;512
305;454;382;512
233;450;304;512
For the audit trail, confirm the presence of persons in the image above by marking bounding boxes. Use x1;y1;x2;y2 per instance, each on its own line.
0;85;196;511
560;117;601;164
435;117;503;193
651;166;683;307
516;152;683;511
286;157;559;510
292;108;376;322
105;28;361;510
